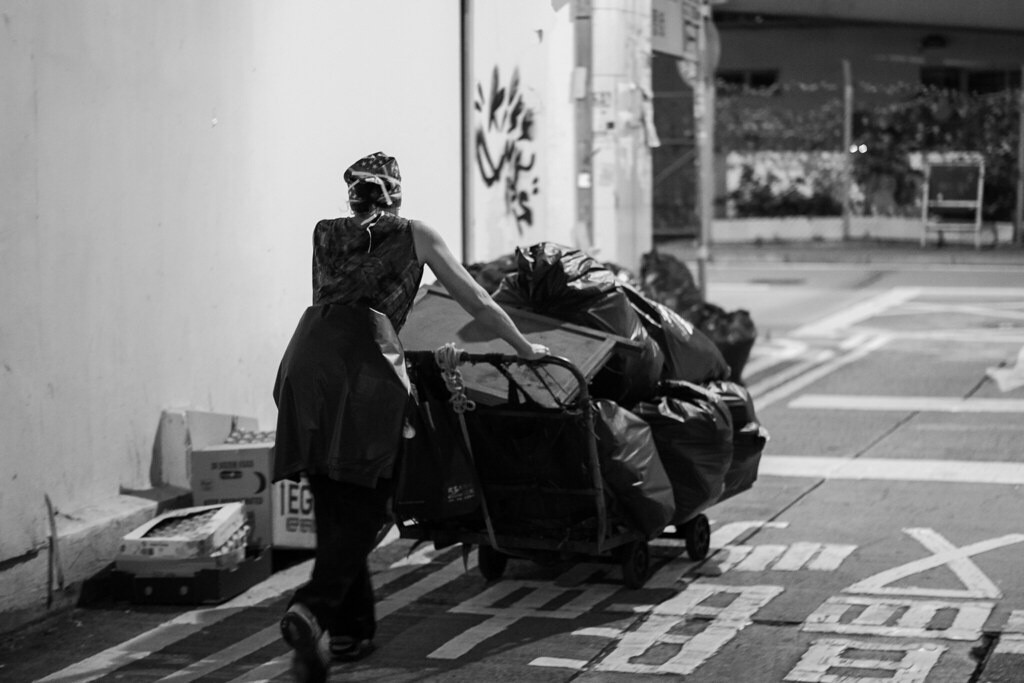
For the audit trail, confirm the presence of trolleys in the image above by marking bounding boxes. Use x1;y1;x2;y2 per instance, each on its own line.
395;350;712;582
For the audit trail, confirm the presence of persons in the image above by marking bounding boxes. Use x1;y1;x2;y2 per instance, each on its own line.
272;151;551;683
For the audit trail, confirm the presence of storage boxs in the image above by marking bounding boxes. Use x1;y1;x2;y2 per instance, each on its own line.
187;410;319;551
117;503;248;571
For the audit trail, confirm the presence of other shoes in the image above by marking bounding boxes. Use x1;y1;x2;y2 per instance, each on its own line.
281;601;329;683
330;637;372;658
